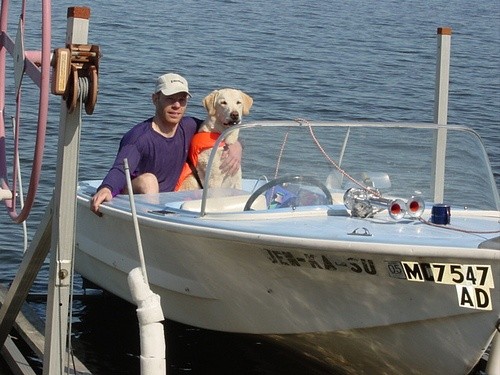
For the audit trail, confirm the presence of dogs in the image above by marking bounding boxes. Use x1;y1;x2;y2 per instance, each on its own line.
175;88;254;190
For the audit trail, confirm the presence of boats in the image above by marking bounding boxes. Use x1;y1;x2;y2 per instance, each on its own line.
71;122;500;375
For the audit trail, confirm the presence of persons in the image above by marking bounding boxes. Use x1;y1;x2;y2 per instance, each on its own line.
89;73;243;219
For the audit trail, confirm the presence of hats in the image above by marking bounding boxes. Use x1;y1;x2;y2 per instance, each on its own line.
155;73;193;98
